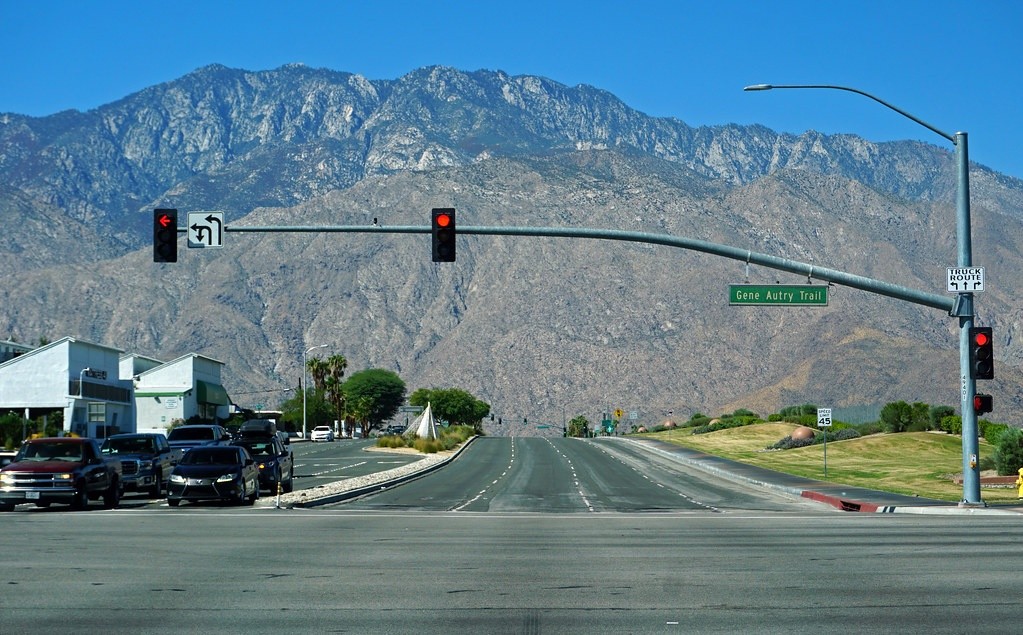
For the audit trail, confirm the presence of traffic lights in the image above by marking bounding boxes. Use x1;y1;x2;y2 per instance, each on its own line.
154;208;177;262
432;208;455;262
974;394;993;414
490;414;494;421
498;418;502;424
523;418;527;425
968;327;994;380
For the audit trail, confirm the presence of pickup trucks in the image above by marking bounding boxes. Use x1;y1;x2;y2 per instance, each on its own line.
0;438;123;517
99;431;183;498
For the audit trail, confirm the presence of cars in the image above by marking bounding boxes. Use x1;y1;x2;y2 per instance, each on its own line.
376;428;393;438
229;438;295;492
310;425;335;442
166;446;260;509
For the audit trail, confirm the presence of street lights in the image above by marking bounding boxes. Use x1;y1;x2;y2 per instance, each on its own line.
746;83;984;507
301;345;329;439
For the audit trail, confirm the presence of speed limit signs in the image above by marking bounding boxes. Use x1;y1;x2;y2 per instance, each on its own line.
817;407;832;427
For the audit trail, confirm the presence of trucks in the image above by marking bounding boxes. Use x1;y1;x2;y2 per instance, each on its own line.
237;420;290;447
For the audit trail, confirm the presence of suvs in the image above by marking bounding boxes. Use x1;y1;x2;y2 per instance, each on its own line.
166;424;231;463
390;425;406;436
